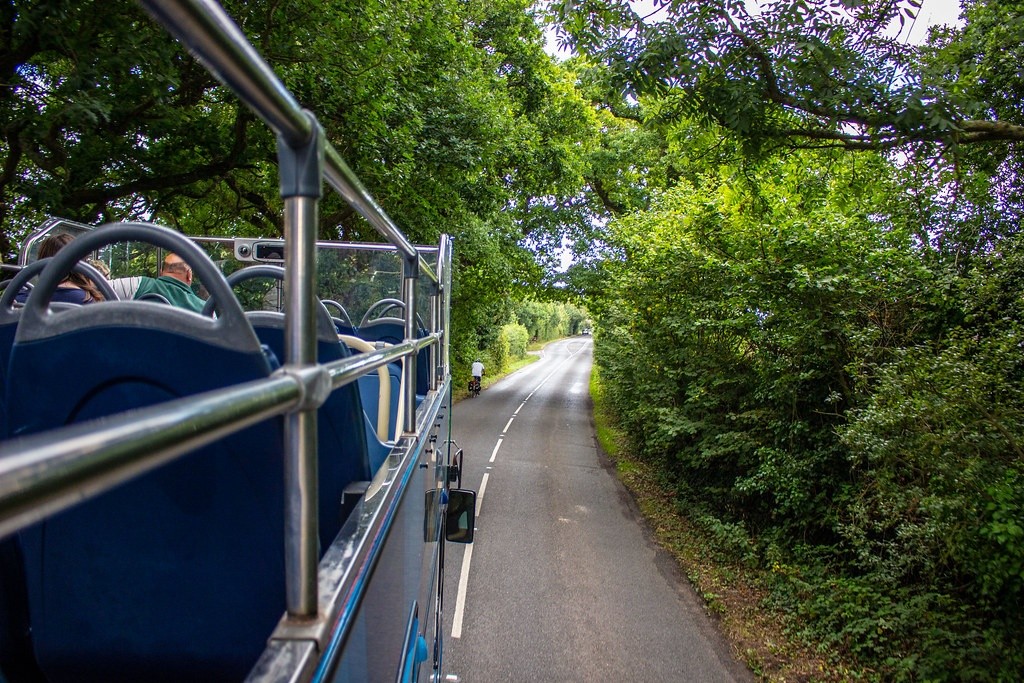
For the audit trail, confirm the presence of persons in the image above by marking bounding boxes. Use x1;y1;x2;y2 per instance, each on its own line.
86;258;111;280
107;253;217;319
0;234;10;263
471;358;486;382
14;232;104;306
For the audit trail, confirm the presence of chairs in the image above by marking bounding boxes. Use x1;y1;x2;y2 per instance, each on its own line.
0;222;436;683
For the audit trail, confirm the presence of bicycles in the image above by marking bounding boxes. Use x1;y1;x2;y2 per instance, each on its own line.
470;372;486;399
